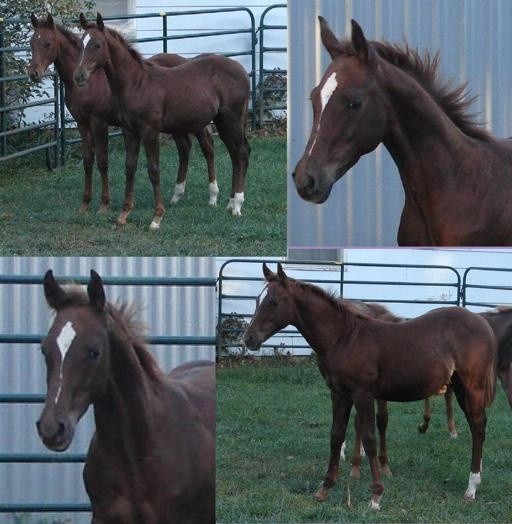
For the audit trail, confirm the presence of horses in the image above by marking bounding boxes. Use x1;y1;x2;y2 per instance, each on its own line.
34;267;216;524
27;11;220;215
72;11;251;230
291;15;512;247
242;260;498;513
475;305;512;412
334;297;460;480
